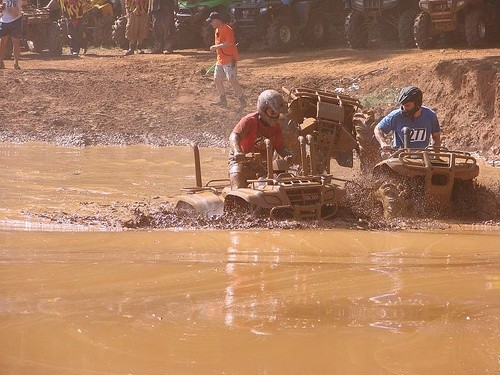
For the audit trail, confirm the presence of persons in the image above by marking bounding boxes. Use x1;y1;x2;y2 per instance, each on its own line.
373;86;441;161
228;89;295;191
126;0;148;55
30;0;114;54
206;12;247;109
148;0;175;54
0;0;27;70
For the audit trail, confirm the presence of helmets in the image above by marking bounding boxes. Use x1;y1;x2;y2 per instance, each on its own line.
257;89;284;127
396;86;423;116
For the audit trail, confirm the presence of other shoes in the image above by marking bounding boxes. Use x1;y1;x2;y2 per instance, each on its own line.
210;95;227;107
236;97;247;113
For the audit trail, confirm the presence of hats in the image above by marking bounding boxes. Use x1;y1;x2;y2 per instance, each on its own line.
206;12;221;22
